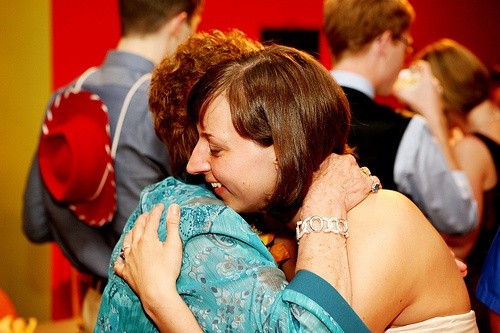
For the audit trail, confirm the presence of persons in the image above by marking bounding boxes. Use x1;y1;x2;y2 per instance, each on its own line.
321;0;481;236
114;42;478;333
410;36;500;332
92;29;384;333
20;0;235;333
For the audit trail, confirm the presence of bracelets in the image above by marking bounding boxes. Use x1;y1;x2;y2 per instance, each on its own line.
294;214;351;240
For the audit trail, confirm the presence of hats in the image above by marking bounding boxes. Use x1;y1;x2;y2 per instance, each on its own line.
37;89;118;227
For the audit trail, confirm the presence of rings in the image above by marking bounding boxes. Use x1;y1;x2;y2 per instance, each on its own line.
360;166;372;175
369;175;380;193
119;243;130;255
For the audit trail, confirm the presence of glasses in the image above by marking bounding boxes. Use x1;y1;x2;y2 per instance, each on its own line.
397;35;414;55
